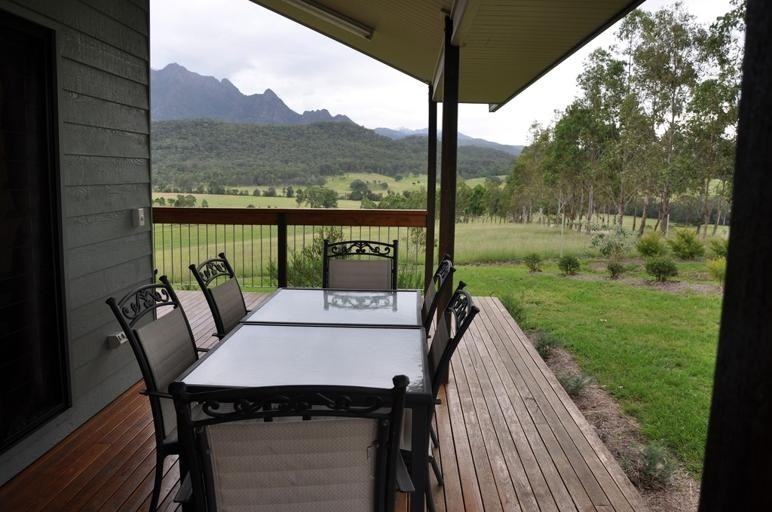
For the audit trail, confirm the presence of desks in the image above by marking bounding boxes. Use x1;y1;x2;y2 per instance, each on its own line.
238;285;424;327
166;322;434;511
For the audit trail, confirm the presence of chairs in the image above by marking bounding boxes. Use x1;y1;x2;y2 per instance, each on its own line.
321;239;399;289
105;253;252;510
169;375;411;511
390;251;480;487
